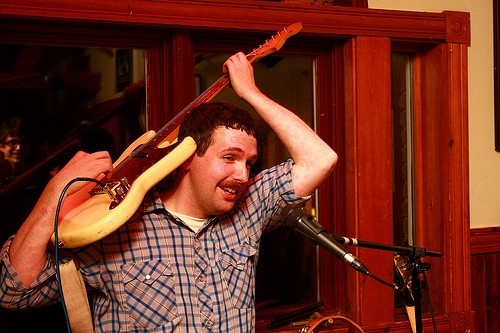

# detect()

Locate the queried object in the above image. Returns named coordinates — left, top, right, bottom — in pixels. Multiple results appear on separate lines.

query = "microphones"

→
left=280, top=204, right=373, bottom=277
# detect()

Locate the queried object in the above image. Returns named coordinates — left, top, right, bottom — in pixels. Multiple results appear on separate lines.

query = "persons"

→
left=1, top=117, right=28, bottom=163
left=0, top=52, right=338, bottom=333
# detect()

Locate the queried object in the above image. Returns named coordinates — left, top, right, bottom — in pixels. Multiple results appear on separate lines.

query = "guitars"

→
left=49, top=21, right=303, bottom=249
